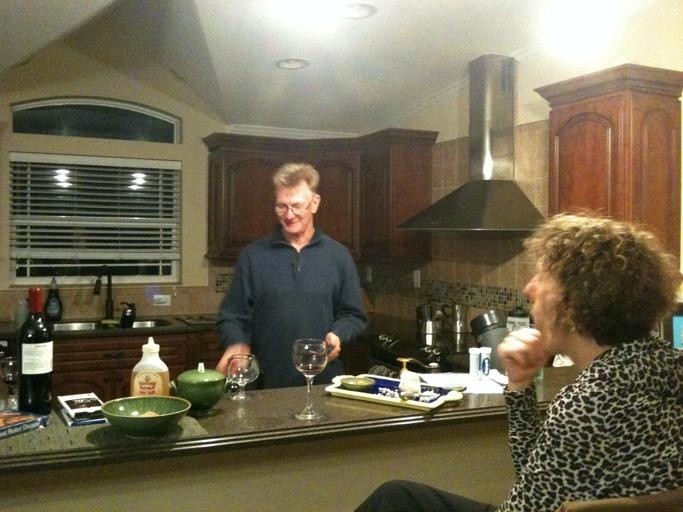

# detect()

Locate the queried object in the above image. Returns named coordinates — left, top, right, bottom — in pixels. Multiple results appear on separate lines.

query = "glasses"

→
left=274, top=195, right=315, bottom=215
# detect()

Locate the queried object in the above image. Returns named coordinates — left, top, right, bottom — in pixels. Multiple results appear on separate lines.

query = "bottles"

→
left=468, top=346, right=492, bottom=376
left=18, top=287, right=54, bottom=415
left=130, top=336, right=170, bottom=397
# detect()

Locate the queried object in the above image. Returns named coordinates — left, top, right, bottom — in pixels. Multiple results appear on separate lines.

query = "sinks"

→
left=52, top=320, right=110, bottom=331
left=107, top=319, right=172, bottom=329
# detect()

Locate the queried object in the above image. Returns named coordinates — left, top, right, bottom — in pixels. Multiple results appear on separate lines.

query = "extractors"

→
left=394, top=54, right=549, bottom=231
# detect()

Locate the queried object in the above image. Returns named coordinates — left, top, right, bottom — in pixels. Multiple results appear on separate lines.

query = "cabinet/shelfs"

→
left=203, top=127, right=438, bottom=264
left=534, top=63, right=683, bottom=275
left=52, top=332, right=221, bottom=404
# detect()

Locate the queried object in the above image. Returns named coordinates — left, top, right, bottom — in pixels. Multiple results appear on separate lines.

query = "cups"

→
left=0, top=356, right=20, bottom=386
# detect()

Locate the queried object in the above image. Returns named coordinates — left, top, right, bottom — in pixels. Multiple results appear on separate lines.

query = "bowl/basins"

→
left=340, top=377, right=376, bottom=391
left=101, top=395, right=192, bottom=439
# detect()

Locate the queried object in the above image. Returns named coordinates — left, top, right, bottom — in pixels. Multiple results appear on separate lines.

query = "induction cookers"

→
left=363, top=323, right=480, bottom=380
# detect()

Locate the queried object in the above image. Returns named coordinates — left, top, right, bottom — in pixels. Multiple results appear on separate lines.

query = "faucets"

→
left=92, top=265, right=114, bottom=319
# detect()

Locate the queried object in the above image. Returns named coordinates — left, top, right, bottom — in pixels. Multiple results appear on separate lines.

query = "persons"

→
left=353, top=210, right=683, bottom=512
left=215, top=162, right=370, bottom=391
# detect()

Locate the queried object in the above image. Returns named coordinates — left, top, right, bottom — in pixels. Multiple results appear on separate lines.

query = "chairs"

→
left=564, top=487, right=683, bottom=512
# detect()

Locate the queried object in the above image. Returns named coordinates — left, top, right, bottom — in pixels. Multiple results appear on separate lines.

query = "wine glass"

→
left=226, top=353, right=260, bottom=401
left=291, top=338, right=329, bottom=422
